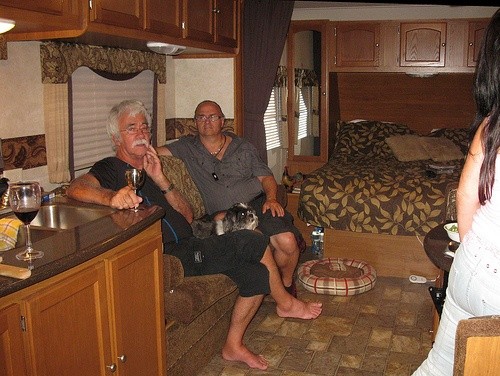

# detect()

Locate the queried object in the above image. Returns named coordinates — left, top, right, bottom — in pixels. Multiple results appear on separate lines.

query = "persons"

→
left=66, top=99, right=323, bottom=370
left=146, top=100, right=306, bottom=301
left=410, top=9, right=500, bottom=376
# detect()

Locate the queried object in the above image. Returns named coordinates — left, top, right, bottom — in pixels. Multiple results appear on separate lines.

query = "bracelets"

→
left=162, top=184, right=176, bottom=196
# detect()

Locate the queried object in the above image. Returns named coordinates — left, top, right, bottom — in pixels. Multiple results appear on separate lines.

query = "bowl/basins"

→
left=444, top=223, right=461, bottom=243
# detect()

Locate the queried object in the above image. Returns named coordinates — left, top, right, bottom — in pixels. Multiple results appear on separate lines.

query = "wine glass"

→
left=8, top=181, right=44, bottom=261
left=124, top=168, right=147, bottom=212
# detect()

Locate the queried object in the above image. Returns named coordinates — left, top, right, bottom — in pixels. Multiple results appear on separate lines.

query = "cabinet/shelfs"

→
left=0, top=194, right=171, bottom=376
left=1, top=1, right=240, bottom=60
left=326, top=17, right=494, bottom=73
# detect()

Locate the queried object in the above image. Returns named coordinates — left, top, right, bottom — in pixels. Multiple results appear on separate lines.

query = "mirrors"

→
left=288, top=18, right=330, bottom=162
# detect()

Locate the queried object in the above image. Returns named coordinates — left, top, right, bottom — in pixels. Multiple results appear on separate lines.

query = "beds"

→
left=297, top=72, right=471, bottom=276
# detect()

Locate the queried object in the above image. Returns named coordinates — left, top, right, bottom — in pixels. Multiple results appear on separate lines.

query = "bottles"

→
left=310, top=226, right=324, bottom=259
left=281, top=167, right=290, bottom=188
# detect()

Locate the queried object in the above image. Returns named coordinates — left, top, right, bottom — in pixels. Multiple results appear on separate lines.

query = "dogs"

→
left=189, top=202, right=259, bottom=239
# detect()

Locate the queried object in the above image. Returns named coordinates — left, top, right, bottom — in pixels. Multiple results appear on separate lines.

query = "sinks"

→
left=0, top=222, right=60, bottom=259
left=1, top=201, right=116, bottom=230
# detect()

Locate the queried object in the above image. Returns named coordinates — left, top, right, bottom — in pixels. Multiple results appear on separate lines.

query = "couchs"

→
left=158, top=245, right=238, bottom=376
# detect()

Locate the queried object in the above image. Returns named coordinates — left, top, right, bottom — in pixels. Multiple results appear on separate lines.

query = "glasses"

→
left=119, top=125, right=152, bottom=135
left=194, top=115, right=221, bottom=122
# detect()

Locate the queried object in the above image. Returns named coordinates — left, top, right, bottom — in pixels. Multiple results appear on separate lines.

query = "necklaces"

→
left=201, top=135, right=227, bottom=160
